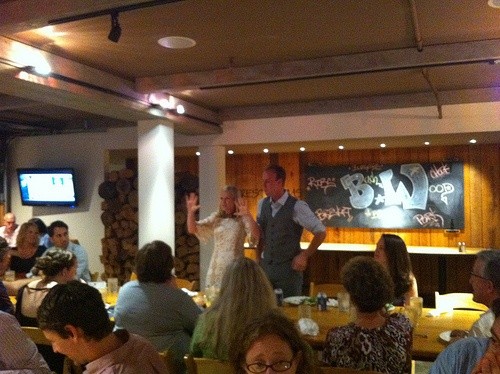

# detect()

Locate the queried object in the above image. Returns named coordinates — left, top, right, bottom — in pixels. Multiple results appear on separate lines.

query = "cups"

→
left=337, top=293, right=350, bottom=313
left=409, top=297, right=423, bottom=317
left=459, top=241, right=466, bottom=252
left=403, top=305, right=420, bottom=327
left=5, top=271, right=15, bottom=282
left=107, top=278, right=118, bottom=294
left=298, top=305, right=311, bottom=320
left=203, top=287, right=219, bottom=308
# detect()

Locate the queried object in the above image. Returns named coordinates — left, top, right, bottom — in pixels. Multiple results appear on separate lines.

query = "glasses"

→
left=468, top=269, right=489, bottom=282
left=242, top=355, right=296, bottom=374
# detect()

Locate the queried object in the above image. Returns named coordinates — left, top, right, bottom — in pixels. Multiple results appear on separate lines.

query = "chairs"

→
left=18, top=271, right=492, bottom=374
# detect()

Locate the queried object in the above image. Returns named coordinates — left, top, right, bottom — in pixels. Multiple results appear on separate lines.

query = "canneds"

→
left=459, top=242, right=466, bottom=252
left=317, top=292, right=327, bottom=311
left=274, top=288, right=283, bottom=306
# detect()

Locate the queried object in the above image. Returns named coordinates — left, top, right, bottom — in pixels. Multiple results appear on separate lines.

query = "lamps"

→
left=108, top=14, right=125, bottom=44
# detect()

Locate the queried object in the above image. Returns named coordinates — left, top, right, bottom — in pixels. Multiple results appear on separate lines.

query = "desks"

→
left=3, top=275, right=209, bottom=323
left=250, top=241, right=489, bottom=292
left=200, top=293, right=488, bottom=361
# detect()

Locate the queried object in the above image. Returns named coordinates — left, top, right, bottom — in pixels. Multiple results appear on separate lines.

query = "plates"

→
left=440, top=331, right=469, bottom=342
left=283, top=296, right=310, bottom=305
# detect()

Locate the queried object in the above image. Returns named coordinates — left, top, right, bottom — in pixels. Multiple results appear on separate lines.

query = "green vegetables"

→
left=301, top=296, right=318, bottom=306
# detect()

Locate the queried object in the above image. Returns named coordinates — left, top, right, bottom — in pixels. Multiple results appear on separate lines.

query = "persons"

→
left=36, top=280, right=172, bottom=374
left=321, top=255, right=413, bottom=374
left=113, top=240, right=204, bottom=358
left=374, top=234, right=419, bottom=307
left=185, top=184, right=261, bottom=299
left=0, top=213, right=92, bottom=374
left=255, top=164, right=327, bottom=299
left=229, top=317, right=324, bottom=374
left=187, top=257, right=281, bottom=364
left=425, top=249, right=500, bottom=374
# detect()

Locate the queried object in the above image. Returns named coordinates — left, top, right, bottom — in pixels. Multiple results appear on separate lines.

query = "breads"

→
left=449, top=329, right=467, bottom=343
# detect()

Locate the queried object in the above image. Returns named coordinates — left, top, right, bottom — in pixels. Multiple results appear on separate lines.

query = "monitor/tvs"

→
left=16, top=167, right=78, bottom=207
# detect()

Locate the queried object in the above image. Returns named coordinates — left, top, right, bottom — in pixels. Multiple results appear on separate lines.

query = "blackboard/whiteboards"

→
left=305, top=159, right=465, bottom=229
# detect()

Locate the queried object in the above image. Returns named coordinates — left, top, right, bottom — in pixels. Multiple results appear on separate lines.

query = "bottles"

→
left=317, top=292, right=328, bottom=311
left=274, top=288, right=283, bottom=307
